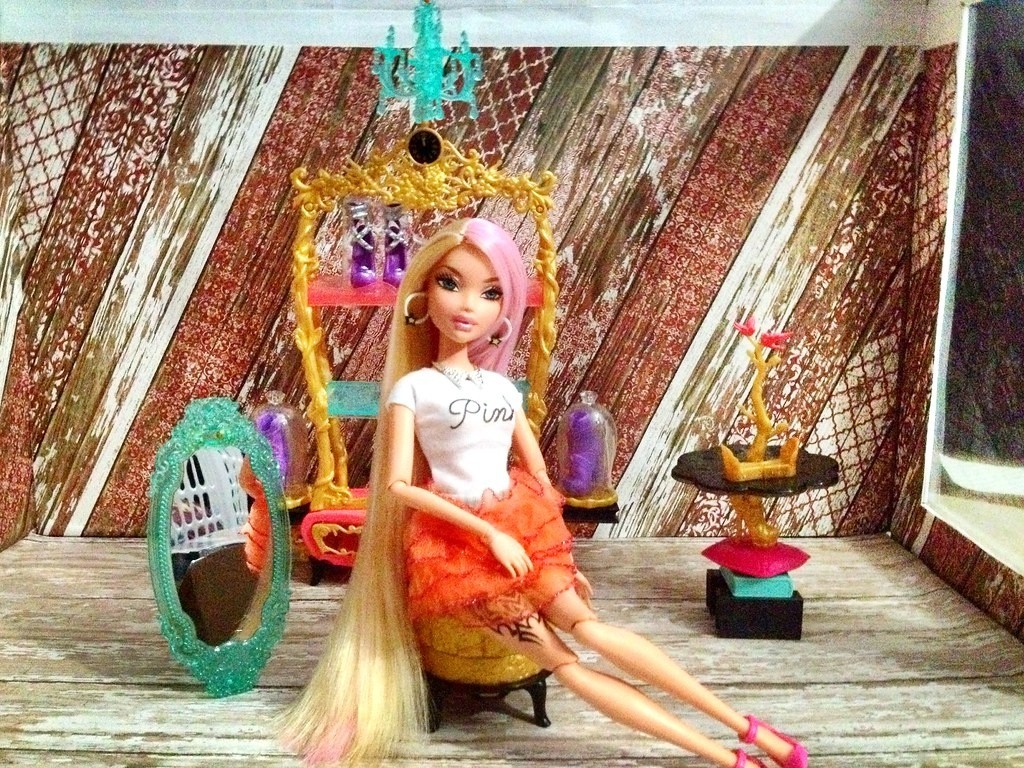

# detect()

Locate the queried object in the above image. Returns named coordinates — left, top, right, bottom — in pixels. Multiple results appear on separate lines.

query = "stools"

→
left=411, top=614, right=557, bottom=732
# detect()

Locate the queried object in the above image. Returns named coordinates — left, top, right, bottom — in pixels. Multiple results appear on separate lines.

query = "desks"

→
left=670, top=445, right=839, bottom=639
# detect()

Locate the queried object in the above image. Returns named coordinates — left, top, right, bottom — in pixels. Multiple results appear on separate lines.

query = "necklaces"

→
left=431, top=360, right=484, bottom=389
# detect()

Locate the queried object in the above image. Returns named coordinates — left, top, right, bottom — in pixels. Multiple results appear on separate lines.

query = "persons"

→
left=270, top=217, right=807, bottom=768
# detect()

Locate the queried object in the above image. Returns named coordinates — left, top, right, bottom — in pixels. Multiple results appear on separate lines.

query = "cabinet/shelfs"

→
left=292, top=126, right=566, bottom=585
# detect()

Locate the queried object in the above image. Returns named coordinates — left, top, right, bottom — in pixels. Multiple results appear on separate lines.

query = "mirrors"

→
left=139, top=393, right=301, bottom=702
